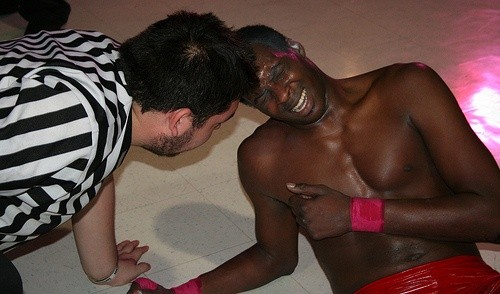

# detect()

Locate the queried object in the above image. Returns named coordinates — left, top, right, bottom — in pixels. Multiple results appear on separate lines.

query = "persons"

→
left=0, top=9, right=263, bottom=294
left=125, top=22, right=499, bottom=293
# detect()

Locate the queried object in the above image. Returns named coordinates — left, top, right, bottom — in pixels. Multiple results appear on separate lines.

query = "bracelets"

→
left=349, top=196, right=385, bottom=234
left=86, top=262, right=120, bottom=286
left=169, top=277, right=203, bottom=293
